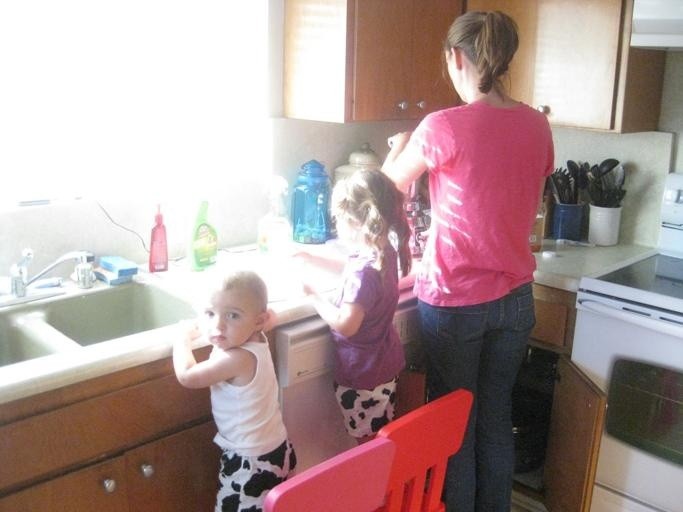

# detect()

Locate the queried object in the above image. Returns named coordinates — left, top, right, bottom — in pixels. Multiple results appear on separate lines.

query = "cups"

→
left=549, top=195, right=586, bottom=249
left=586, top=196, right=624, bottom=249
left=148, top=203, right=169, bottom=274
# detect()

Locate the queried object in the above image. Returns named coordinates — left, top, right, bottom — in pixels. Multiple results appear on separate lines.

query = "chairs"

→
left=375, top=388, right=474, bottom=512
left=262, top=437, right=395, bottom=512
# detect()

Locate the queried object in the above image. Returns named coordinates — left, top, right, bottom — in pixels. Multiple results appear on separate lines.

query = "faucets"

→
left=10, top=248, right=95, bottom=297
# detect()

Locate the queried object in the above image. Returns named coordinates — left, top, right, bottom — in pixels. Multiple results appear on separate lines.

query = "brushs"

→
left=556, top=239, right=596, bottom=248
left=615, top=165, right=625, bottom=206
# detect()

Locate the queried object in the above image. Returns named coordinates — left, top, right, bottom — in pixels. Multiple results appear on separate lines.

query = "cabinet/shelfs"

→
left=284, top=0, right=466, bottom=123
left=0, top=331, right=280, bottom=512
left=509, top=284, right=611, bottom=512
left=466, top=0, right=667, bottom=133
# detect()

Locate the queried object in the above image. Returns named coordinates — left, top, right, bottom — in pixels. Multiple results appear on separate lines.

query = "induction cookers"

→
left=578, top=246, right=682, bottom=323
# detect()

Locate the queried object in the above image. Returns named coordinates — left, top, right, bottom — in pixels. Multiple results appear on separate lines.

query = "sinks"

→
left=0, top=311, right=83, bottom=408
left=41, top=282, right=223, bottom=379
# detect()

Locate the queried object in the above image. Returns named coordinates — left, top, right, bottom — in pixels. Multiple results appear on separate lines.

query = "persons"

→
left=377, top=8, right=558, bottom=511
left=171, top=270, right=298, bottom=512
left=294, top=165, right=414, bottom=446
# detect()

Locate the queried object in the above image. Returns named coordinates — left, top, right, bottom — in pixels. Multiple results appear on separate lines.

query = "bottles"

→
left=191, top=201, right=218, bottom=271
left=333, top=142, right=387, bottom=187
left=289, top=152, right=332, bottom=247
left=526, top=201, right=548, bottom=253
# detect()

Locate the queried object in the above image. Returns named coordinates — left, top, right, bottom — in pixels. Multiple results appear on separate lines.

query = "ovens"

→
left=570, top=292, right=682, bottom=511
